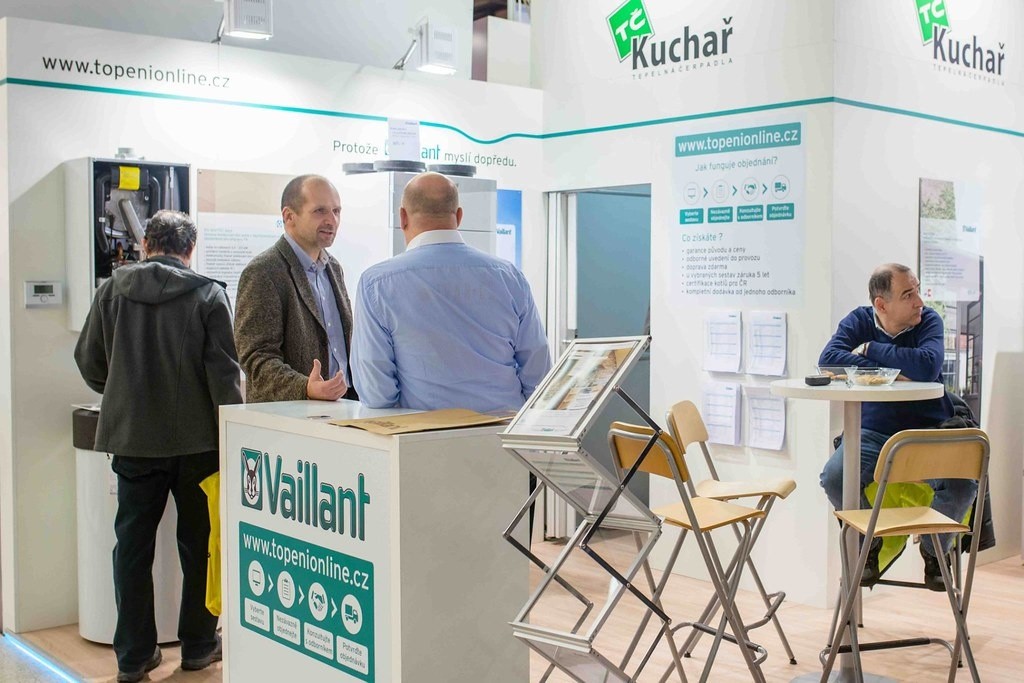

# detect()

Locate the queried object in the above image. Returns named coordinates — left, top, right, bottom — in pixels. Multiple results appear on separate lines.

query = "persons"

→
left=72, top=207, right=244, bottom=683
left=349, top=169, right=552, bottom=552
left=819, top=264, right=976, bottom=592
left=231, top=173, right=354, bottom=402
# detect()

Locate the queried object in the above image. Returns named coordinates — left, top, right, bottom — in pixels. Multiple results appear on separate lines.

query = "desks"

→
left=771, top=377, right=945, bottom=683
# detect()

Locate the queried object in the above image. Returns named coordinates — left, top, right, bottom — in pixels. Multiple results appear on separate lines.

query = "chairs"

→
left=663, top=399, right=797, bottom=683
left=607, top=423, right=768, bottom=683
left=818, top=427, right=991, bottom=683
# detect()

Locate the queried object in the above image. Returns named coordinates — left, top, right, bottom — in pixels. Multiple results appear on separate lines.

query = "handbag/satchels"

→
left=198, top=470, right=222, bottom=617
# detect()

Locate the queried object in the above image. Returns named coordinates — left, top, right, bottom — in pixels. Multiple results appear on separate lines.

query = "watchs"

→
left=857, top=342, right=865, bottom=356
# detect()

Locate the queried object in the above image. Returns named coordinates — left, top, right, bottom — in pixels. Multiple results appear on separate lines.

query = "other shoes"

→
left=117, top=645, right=162, bottom=682
left=181, top=633, right=222, bottom=670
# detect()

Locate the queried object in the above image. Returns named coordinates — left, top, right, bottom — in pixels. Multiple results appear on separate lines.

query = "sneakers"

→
left=860, top=549, right=880, bottom=586
left=920, top=542, right=953, bottom=591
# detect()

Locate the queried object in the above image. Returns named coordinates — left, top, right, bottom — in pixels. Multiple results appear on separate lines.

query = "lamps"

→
left=211, top=0, right=274, bottom=45
left=389, top=14, right=457, bottom=75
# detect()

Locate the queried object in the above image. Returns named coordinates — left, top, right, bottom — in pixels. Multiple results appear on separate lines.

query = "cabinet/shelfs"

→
left=330, top=171, right=497, bottom=307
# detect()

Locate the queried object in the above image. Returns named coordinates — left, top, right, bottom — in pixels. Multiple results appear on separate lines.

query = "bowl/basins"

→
left=844, top=367, right=901, bottom=386
left=814, top=364, right=858, bottom=383
